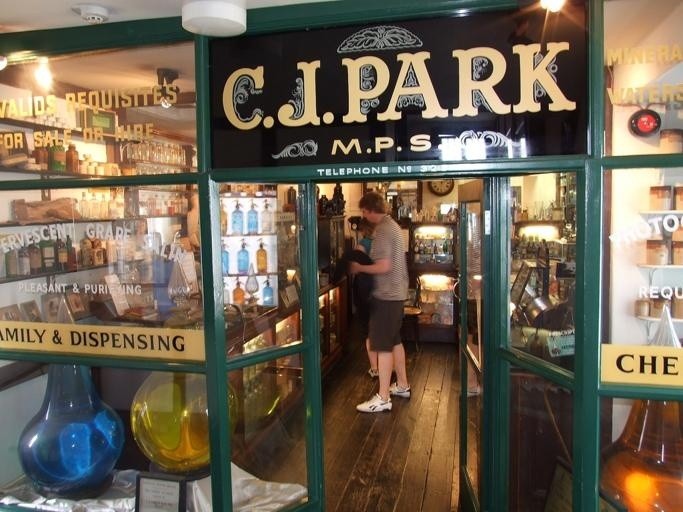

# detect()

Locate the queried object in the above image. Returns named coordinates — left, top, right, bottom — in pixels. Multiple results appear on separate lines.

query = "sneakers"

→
left=355, top=392, right=393, bottom=414
left=367, top=367, right=379, bottom=379
left=388, top=381, right=411, bottom=399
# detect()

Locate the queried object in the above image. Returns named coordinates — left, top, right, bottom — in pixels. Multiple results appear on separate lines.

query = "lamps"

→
left=182, top=2, right=246, bottom=36
left=154, top=68, right=179, bottom=110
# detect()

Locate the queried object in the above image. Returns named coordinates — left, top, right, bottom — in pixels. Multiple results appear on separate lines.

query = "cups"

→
left=557, top=282, right=570, bottom=301
left=135, top=164, right=181, bottom=175
left=634, top=128, right=683, bottom=320
left=77, top=159, right=120, bottom=177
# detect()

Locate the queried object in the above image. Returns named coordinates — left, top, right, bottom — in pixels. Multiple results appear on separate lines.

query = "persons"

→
left=348, top=192, right=413, bottom=414
left=338, top=217, right=381, bottom=380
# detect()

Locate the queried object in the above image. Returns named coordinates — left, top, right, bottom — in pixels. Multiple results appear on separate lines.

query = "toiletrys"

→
left=218, top=196, right=275, bottom=307
left=511, top=198, right=562, bottom=265
left=409, top=202, right=454, bottom=264
left=4, top=137, right=191, bottom=281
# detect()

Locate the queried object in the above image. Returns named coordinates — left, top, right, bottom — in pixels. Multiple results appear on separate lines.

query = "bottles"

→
left=409, top=203, right=457, bottom=222
left=5, top=231, right=72, bottom=279
left=80, top=231, right=106, bottom=266
left=512, top=199, right=551, bottom=221
left=126, top=137, right=185, bottom=165
left=135, top=191, right=191, bottom=217
left=512, top=233, right=560, bottom=258
left=65, top=144, right=79, bottom=174
left=414, top=240, right=454, bottom=257
left=78, top=190, right=123, bottom=220
